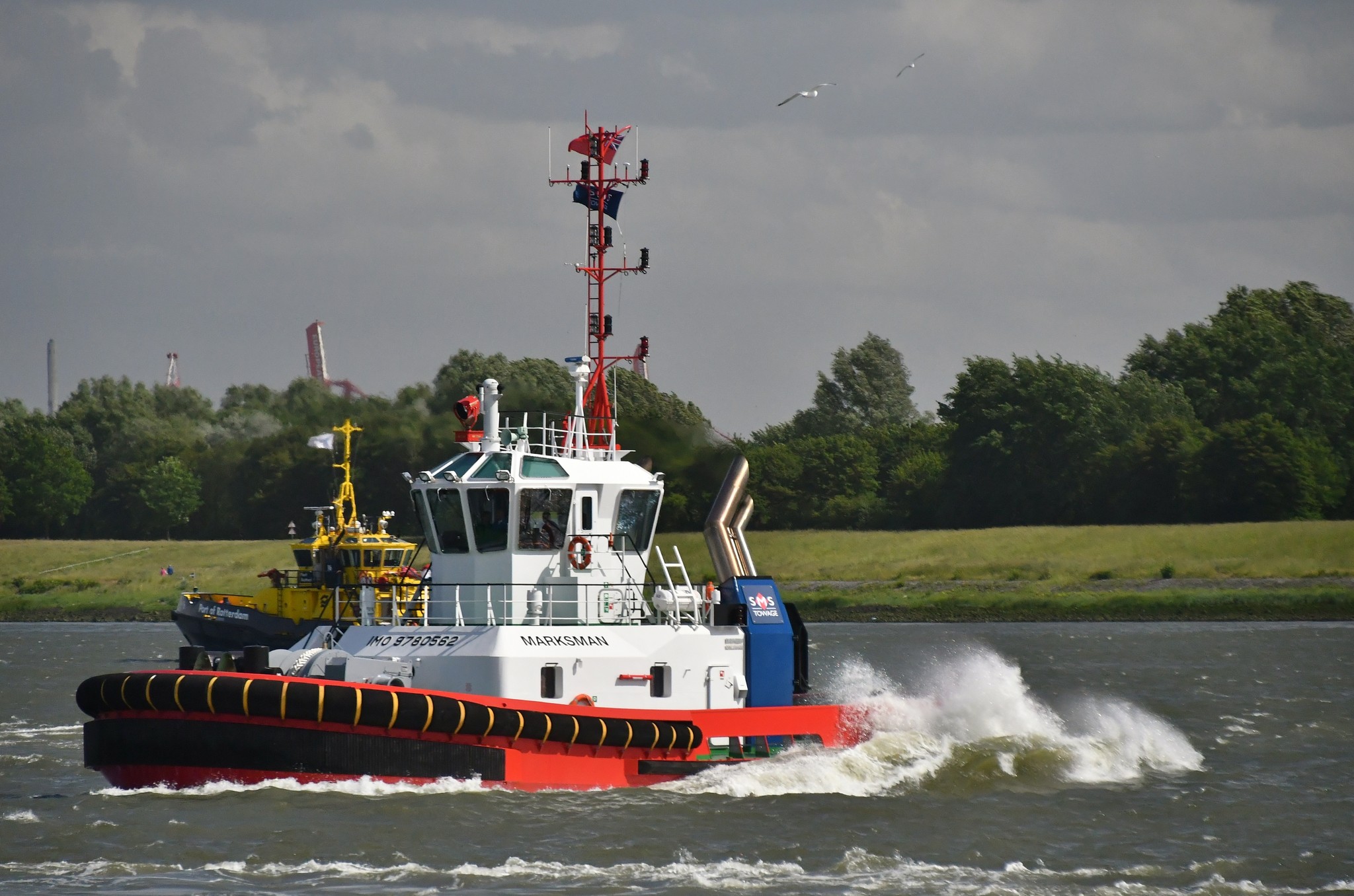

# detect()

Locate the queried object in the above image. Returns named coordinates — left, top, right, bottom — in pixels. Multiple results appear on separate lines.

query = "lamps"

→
left=653, top=472, right=666, bottom=482
left=640, top=247, right=649, bottom=268
left=444, top=471, right=461, bottom=483
left=589, top=315, right=599, bottom=333
left=418, top=471, right=433, bottom=482
left=312, top=510, right=323, bottom=535
left=604, top=225, right=613, bottom=245
left=401, top=472, right=414, bottom=483
left=603, top=314, right=613, bottom=334
left=640, top=158, right=649, bottom=177
left=590, top=135, right=598, bottom=156
left=589, top=225, right=599, bottom=245
left=495, top=469, right=514, bottom=482
left=453, top=395, right=484, bottom=442
left=581, top=160, right=588, bottom=178
left=640, top=335, right=649, bottom=355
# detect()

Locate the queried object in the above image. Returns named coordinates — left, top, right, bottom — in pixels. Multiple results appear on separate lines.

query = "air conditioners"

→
left=378, top=520, right=388, bottom=534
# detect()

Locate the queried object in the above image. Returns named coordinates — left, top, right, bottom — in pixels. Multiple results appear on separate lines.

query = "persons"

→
left=167, top=565, right=173, bottom=575
left=160, top=567, right=166, bottom=576
left=541, top=512, right=560, bottom=541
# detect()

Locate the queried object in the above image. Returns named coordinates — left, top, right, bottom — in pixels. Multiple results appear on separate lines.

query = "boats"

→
left=169, top=412, right=428, bottom=648
left=74, top=104, right=872, bottom=787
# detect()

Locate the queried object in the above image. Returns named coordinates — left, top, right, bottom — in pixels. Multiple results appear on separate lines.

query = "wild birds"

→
left=896, top=51, right=926, bottom=79
left=776, top=81, right=839, bottom=108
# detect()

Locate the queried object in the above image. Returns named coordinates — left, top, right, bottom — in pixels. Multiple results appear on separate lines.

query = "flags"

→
left=307, top=433, right=335, bottom=450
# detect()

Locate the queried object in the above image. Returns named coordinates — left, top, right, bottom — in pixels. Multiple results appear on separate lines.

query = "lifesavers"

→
left=570, top=694, right=594, bottom=707
left=378, top=576, right=389, bottom=584
left=706, top=581, right=714, bottom=611
left=359, top=570, right=367, bottom=583
left=568, top=537, right=591, bottom=569
left=409, top=622, right=417, bottom=626
